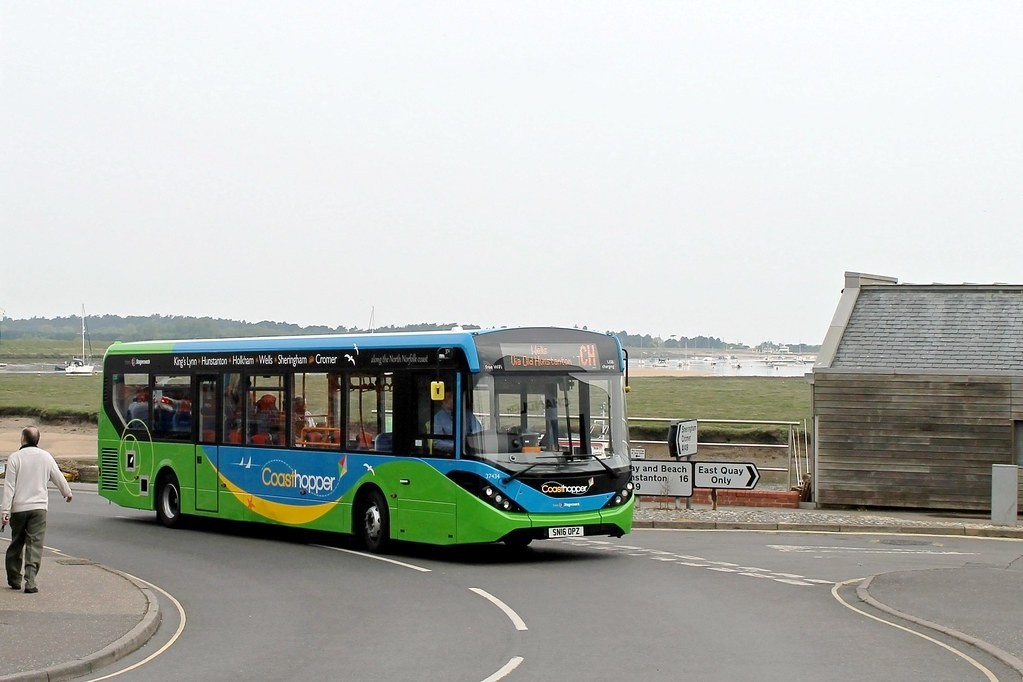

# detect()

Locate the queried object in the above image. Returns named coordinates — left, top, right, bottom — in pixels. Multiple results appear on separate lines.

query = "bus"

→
left=97, top=324, right=636, bottom=555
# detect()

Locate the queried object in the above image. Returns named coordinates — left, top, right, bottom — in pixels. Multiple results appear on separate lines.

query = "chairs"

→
left=326, top=432, right=340, bottom=448
left=249, top=432, right=273, bottom=445
left=305, top=432, right=323, bottom=449
left=203, top=429, right=216, bottom=441
left=356, top=431, right=374, bottom=450
left=226, top=431, right=241, bottom=444
left=154, top=403, right=173, bottom=429
left=173, top=399, right=191, bottom=432
left=374, top=433, right=392, bottom=452
left=131, top=402, right=149, bottom=430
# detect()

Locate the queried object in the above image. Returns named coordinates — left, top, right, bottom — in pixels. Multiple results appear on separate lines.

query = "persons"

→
left=434, top=387, right=482, bottom=453
left=2, top=426, right=72, bottom=593
left=293, top=396, right=316, bottom=447
left=225, top=379, right=281, bottom=445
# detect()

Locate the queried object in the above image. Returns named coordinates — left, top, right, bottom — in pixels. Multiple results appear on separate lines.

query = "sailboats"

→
left=63, top=303, right=104, bottom=376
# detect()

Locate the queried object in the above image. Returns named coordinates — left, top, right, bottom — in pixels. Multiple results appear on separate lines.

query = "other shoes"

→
left=24, top=586, right=39, bottom=593
left=12, top=585, right=21, bottom=589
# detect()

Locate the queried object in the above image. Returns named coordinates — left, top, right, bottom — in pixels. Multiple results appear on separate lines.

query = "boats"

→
left=635, top=332, right=817, bottom=372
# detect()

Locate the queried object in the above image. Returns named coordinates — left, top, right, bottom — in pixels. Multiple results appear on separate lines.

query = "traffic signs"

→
left=676, top=420, right=698, bottom=458
left=694, top=460, right=760, bottom=489
